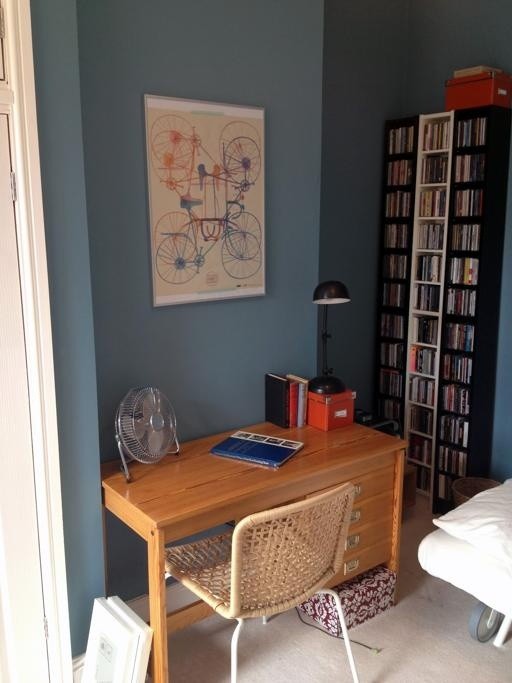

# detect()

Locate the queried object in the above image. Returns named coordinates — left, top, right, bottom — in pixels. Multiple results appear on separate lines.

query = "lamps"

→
left=307, top=280, right=352, bottom=393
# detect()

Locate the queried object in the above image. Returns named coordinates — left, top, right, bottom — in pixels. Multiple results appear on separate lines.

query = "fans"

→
left=113, top=385, right=180, bottom=483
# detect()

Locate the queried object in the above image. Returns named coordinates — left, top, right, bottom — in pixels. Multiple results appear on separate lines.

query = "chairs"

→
left=165, top=479, right=360, bottom=683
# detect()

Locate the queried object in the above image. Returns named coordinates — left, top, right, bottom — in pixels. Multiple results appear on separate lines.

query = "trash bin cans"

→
left=450, top=477, right=501, bottom=507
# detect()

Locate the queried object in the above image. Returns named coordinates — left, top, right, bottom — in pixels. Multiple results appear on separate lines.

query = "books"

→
left=264, top=372, right=309, bottom=429
left=208, top=429, right=305, bottom=467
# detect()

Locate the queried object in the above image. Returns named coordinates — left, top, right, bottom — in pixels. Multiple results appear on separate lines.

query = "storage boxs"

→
left=296, top=562, right=398, bottom=637
left=443, top=70, right=511, bottom=111
left=305, top=388, right=357, bottom=432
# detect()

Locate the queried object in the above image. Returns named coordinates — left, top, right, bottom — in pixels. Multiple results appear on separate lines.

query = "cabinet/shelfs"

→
left=369, top=102, right=512, bottom=514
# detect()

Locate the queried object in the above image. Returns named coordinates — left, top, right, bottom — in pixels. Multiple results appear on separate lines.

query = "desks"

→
left=101, top=420, right=408, bottom=683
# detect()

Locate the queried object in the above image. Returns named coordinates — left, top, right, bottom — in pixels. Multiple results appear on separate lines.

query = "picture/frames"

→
left=143, top=93, right=267, bottom=308
